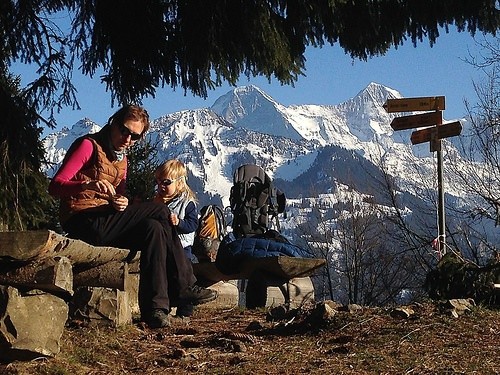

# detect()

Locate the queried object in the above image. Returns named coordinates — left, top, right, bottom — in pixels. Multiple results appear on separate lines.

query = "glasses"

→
left=118, top=123, right=140, bottom=141
left=156, top=176, right=184, bottom=186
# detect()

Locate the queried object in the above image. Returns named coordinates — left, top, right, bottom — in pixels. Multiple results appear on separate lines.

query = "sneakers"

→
left=179, top=286, right=217, bottom=304
left=144, top=309, right=170, bottom=328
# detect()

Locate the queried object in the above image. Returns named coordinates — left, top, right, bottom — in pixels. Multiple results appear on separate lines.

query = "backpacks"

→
left=195, top=205, right=226, bottom=259
left=229, top=164, right=286, bottom=240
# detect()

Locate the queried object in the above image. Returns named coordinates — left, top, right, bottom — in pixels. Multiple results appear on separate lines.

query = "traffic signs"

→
left=390, top=111, right=442, bottom=131
left=382, top=96, right=445, bottom=113
left=410, top=121, right=462, bottom=145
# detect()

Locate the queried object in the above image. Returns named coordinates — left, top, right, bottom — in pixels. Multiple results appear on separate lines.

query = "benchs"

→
left=0, top=230, right=141, bottom=357
left=177, top=256, right=326, bottom=306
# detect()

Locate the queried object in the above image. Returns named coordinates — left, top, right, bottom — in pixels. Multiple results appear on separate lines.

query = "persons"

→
left=48, top=104, right=218, bottom=329
left=153, top=158, right=200, bottom=318
